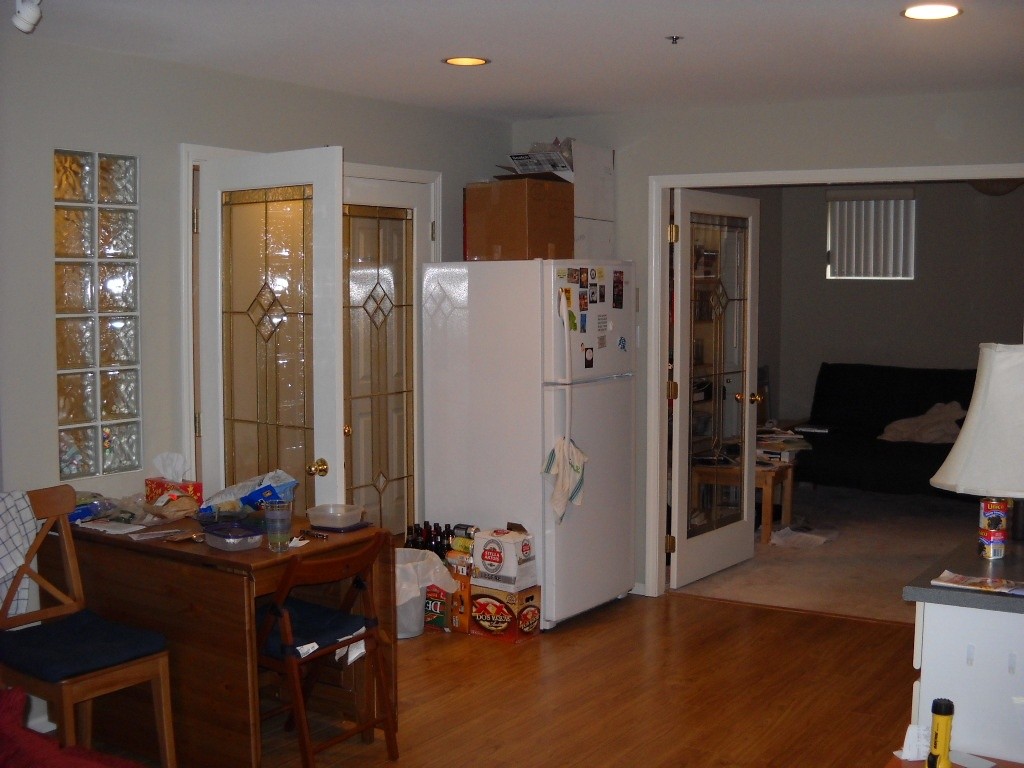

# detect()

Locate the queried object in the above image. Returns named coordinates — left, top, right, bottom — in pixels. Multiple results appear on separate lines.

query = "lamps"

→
left=11, top=0, right=42, bottom=33
left=929, top=343, right=1024, bottom=499
left=966, top=177, right=1024, bottom=196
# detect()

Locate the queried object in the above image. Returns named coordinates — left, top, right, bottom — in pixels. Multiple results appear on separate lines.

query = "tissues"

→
left=144, top=452, right=204, bottom=512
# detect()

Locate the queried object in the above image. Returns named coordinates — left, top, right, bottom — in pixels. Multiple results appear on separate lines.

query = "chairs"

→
left=255, top=530, right=399, bottom=768
left=1, top=485, right=178, bottom=768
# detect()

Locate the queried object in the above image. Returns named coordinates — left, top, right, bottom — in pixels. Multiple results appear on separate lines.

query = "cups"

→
left=264, top=500, right=292, bottom=552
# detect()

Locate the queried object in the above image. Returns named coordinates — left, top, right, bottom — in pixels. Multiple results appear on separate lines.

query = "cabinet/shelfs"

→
left=668, top=223, right=721, bottom=453
left=902, top=538, right=1024, bottom=762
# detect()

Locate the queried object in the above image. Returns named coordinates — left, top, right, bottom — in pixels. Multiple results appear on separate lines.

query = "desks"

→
left=36, top=512, right=396, bottom=768
left=690, top=459, right=799, bottom=544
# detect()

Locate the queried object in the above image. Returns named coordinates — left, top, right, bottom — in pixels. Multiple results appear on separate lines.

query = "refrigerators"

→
left=421, top=257, right=636, bottom=631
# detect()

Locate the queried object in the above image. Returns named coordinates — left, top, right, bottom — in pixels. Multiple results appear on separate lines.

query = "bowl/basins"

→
left=305, top=503, right=365, bottom=528
left=202, top=522, right=264, bottom=551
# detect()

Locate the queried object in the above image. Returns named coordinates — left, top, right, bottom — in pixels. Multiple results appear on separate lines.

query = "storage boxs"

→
left=563, top=141, right=615, bottom=260
left=466, top=172, right=574, bottom=261
left=424, top=522, right=542, bottom=645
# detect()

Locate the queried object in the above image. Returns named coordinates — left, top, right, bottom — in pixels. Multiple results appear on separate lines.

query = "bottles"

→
left=404, top=521, right=455, bottom=561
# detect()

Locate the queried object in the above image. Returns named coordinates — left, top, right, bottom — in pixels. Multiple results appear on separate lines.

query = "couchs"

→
left=785, top=362, right=978, bottom=500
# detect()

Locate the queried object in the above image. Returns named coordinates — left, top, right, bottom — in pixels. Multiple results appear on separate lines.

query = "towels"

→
left=541, top=434, right=588, bottom=528
